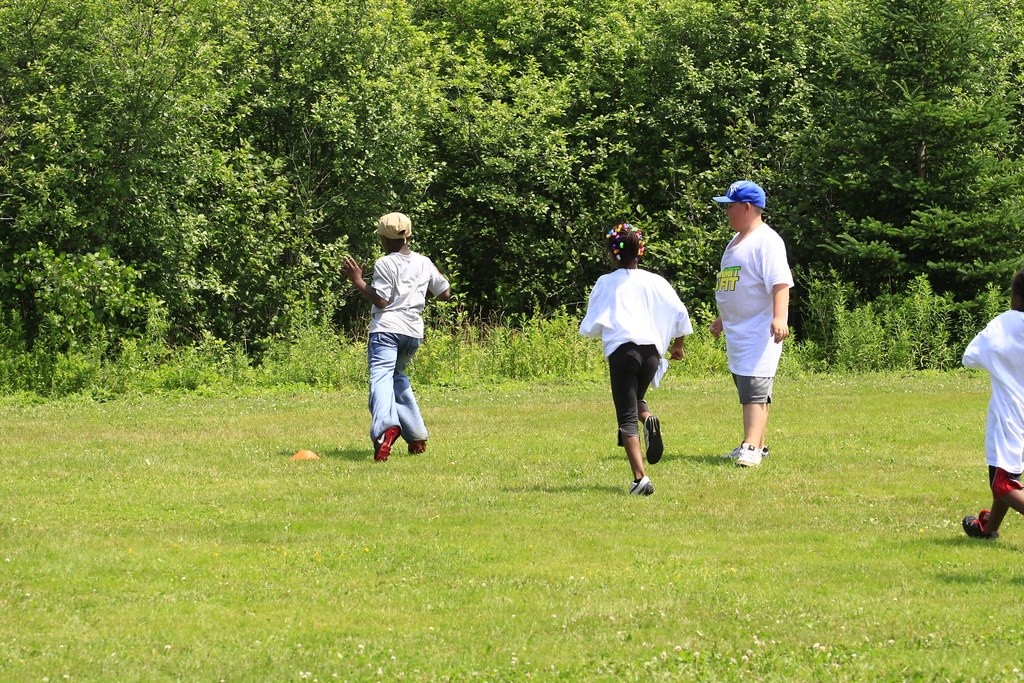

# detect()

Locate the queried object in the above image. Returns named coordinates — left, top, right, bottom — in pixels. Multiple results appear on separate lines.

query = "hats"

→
left=378, top=212, right=412, bottom=239
left=712, top=181, right=767, bottom=209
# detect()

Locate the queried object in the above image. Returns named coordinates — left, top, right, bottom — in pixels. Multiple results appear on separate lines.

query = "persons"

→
left=342, top=212, right=450, bottom=461
left=578, top=223, right=693, bottom=497
left=961, top=267, right=1024, bottom=540
left=707, top=180, right=796, bottom=469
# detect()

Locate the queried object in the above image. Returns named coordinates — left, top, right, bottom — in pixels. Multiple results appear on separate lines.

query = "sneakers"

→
left=644, top=416, right=665, bottom=465
left=373, top=425, right=400, bottom=461
left=628, top=477, right=653, bottom=497
left=720, top=443, right=770, bottom=467
left=408, top=438, right=427, bottom=454
left=963, top=511, right=999, bottom=540
